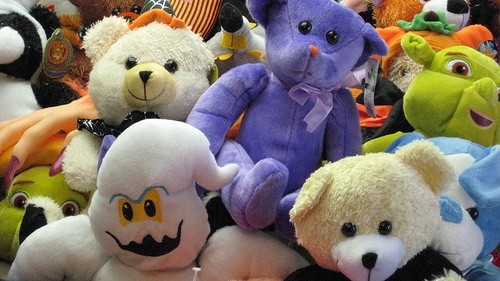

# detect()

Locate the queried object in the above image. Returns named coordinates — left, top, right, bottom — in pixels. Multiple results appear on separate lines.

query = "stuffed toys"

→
left=0, top=0, right=500, bottom=281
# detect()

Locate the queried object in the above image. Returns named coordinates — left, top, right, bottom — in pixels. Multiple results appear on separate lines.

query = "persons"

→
left=0, top=93, right=97, bottom=186
